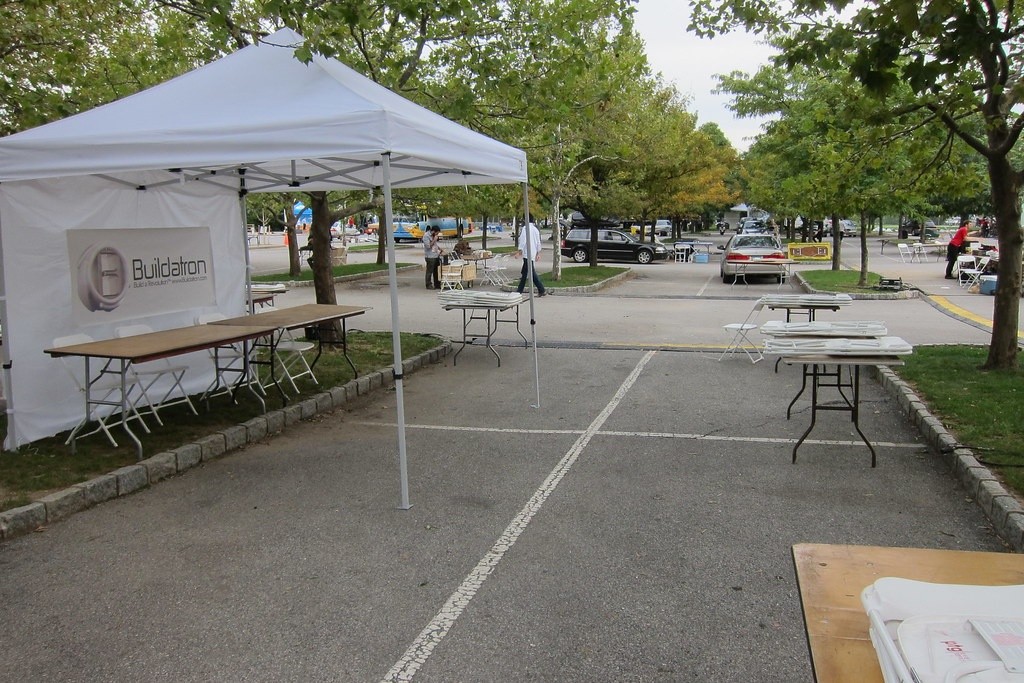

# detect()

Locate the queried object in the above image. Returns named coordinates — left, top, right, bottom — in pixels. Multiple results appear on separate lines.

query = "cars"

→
left=716, top=233, right=787, bottom=284
left=329, top=217, right=381, bottom=238
left=623, top=219, right=674, bottom=238
left=829, top=220, right=857, bottom=237
left=901, top=215, right=938, bottom=236
left=735, top=216, right=783, bottom=247
left=797, top=219, right=832, bottom=237
left=538, top=218, right=564, bottom=228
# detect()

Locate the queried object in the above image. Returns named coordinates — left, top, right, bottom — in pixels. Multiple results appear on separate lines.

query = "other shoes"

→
left=533, top=291, right=546, bottom=297
left=945, top=274, right=957, bottom=279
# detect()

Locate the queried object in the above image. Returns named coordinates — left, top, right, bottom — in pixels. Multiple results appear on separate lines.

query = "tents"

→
left=0, top=26, right=541, bottom=509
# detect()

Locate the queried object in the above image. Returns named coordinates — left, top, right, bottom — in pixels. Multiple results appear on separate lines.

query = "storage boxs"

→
left=438, top=265, right=476, bottom=281
left=695, top=254, right=709, bottom=263
left=980, top=275, right=997, bottom=295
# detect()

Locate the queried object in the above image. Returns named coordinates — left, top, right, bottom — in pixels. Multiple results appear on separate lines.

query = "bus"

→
left=391, top=196, right=473, bottom=244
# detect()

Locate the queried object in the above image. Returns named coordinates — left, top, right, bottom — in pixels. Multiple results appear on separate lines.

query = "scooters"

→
left=978, top=220, right=999, bottom=238
left=718, top=224, right=726, bottom=235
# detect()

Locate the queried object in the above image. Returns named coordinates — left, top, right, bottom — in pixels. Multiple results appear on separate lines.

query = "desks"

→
left=727, top=261, right=800, bottom=295
left=780, top=336, right=905, bottom=468
left=418, top=235, right=451, bottom=248
left=299, top=249, right=312, bottom=265
left=441, top=296, right=530, bottom=367
left=453, top=257, right=493, bottom=288
left=675, top=241, right=713, bottom=263
left=766, top=294, right=851, bottom=373
left=44, top=290, right=373, bottom=458
left=908, top=244, right=948, bottom=263
left=791, top=541, right=1024, bottom=683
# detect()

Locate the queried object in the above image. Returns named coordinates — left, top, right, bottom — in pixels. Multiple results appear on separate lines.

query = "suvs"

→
left=561, top=209, right=669, bottom=264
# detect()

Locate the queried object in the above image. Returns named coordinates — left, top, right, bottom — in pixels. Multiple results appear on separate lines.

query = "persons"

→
left=944, top=220, right=972, bottom=279
left=979, top=216, right=990, bottom=237
left=839, top=218, right=846, bottom=243
left=423, top=225, right=444, bottom=290
left=515, top=212, right=547, bottom=298
left=812, top=222, right=823, bottom=242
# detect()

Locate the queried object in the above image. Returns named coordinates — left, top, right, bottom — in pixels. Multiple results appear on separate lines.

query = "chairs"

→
left=195, top=313, right=267, bottom=404
left=53, top=333, right=151, bottom=447
left=898, top=243, right=928, bottom=263
left=861, top=576, right=1024, bottom=683
left=718, top=297, right=912, bottom=366
left=115, top=324, right=198, bottom=426
left=675, top=245, right=703, bottom=263
left=437, top=289, right=523, bottom=309
left=441, top=260, right=464, bottom=292
left=479, top=254, right=510, bottom=287
left=957, top=256, right=990, bottom=289
left=257, top=307, right=318, bottom=394
